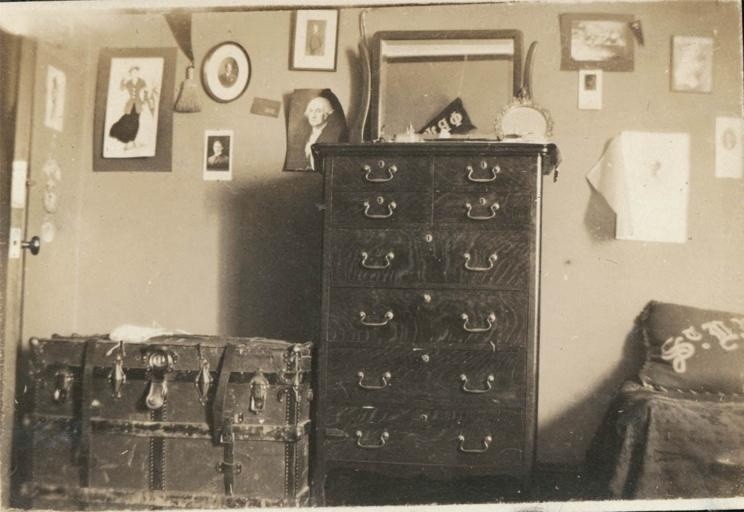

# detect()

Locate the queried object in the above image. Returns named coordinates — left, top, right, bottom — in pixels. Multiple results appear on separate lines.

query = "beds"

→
left=603, top=380, right=744, bottom=497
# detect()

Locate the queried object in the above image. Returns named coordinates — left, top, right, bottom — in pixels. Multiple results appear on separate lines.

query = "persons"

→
left=288, top=97, right=340, bottom=175
left=206, top=139, right=229, bottom=170
left=108, top=64, right=155, bottom=151
left=307, top=23, right=323, bottom=54
left=219, top=62, right=238, bottom=88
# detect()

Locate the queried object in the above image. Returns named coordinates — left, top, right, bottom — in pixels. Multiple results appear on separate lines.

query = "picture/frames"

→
left=199, top=41, right=251, bottom=104
left=288, top=8, right=340, bottom=72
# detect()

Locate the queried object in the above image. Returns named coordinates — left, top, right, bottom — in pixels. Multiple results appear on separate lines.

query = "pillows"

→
left=635, top=299, right=744, bottom=402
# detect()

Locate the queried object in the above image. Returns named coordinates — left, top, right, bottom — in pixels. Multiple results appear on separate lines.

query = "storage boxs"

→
left=8, top=334, right=309, bottom=510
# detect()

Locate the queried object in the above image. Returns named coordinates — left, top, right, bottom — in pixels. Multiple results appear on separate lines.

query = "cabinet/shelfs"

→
left=308, top=143, right=547, bottom=508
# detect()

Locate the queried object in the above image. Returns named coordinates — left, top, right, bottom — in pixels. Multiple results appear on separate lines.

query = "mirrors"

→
left=374, top=30, right=523, bottom=141
